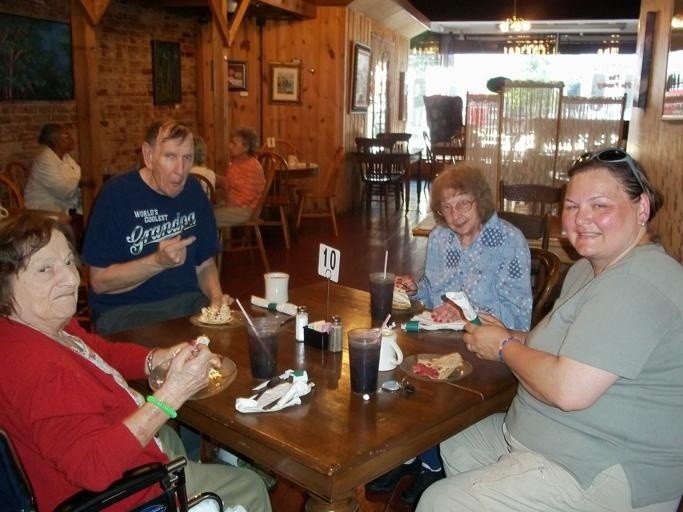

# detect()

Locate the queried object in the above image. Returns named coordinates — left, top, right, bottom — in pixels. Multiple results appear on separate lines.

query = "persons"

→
left=1, top=209, right=272, bottom=511
left=25, top=122, right=94, bottom=231
left=369, top=165, right=534, bottom=502
left=417, top=149, right=683, bottom=512
left=193, top=135, right=216, bottom=200
left=82, top=119, right=275, bottom=492
left=211, top=127, right=268, bottom=229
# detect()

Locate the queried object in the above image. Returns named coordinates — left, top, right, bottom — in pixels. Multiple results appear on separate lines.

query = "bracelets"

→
left=148, top=347, right=158, bottom=371
left=499, top=337, right=514, bottom=364
left=147, top=393, right=177, bottom=421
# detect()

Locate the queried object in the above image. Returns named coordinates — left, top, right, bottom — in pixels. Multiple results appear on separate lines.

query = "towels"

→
left=410, top=310, right=467, bottom=332
left=234, top=368, right=312, bottom=413
left=249, top=295, right=298, bottom=317
left=444, top=291, right=476, bottom=321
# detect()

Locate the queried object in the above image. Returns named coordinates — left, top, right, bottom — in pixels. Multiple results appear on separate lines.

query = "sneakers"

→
left=400, top=464, right=446, bottom=504
left=214, top=447, right=277, bottom=489
left=366, top=457, right=420, bottom=493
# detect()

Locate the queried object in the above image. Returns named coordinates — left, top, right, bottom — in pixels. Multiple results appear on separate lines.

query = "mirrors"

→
left=661, top=0, right=683, bottom=121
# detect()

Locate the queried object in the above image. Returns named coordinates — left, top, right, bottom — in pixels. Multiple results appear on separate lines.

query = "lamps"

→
left=498, top=0, right=532, bottom=35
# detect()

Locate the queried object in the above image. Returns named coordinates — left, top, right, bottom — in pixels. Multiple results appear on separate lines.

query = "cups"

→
left=264, top=273, right=289, bottom=304
left=248, top=317, right=285, bottom=376
left=347, top=329, right=382, bottom=392
left=370, top=271, right=395, bottom=322
left=289, top=155, right=300, bottom=167
left=375, top=326, right=403, bottom=370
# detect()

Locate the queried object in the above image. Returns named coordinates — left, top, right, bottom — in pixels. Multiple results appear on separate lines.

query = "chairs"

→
left=499, top=180, right=568, bottom=237
left=6, top=161, right=28, bottom=210
left=529, top=246, right=561, bottom=330
left=1, top=174, right=76, bottom=248
left=496, top=211, right=552, bottom=252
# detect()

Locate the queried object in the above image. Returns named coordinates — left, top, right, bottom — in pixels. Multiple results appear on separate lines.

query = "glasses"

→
left=571, top=149, right=644, bottom=190
left=436, top=199, right=479, bottom=217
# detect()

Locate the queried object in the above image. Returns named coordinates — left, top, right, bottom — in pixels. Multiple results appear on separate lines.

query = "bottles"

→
left=329, top=317, right=344, bottom=354
left=296, top=305, right=309, bottom=343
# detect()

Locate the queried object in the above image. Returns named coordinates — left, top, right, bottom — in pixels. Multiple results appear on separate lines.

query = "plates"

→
left=191, top=310, right=246, bottom=329
left=391, top=299, right=424, bottom=317
left=147, top=355, right=236, bottom=400
left=400, top=354, right=473, bottom=383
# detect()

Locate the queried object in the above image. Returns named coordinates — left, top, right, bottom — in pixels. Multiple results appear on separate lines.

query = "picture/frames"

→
left=268, top=62, right=302, bottom=105
left=632, top=12, right=657, bottom=110
left=151, top=40, right=182, bottom=105
left=228, top=60, right=247, bottom=92
left=347, top=41, right=374, bottom=113
left=0, top=12, right=75, bottom=102
left=398, top=71, right=409, bottom=122
